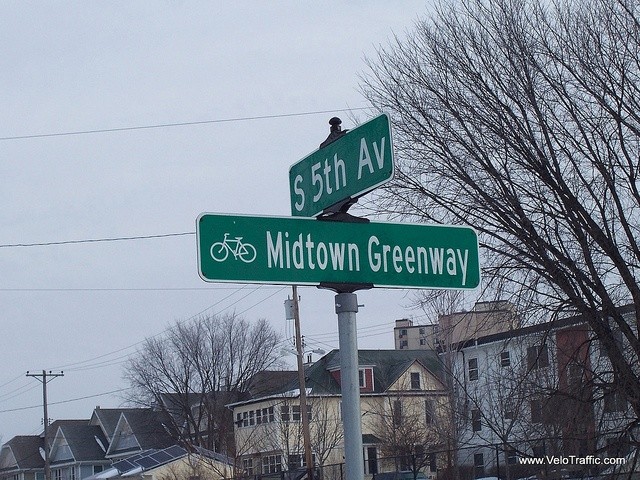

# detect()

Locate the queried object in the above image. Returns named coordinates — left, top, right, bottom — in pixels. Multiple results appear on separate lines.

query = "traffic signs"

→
left=288, top=111, right=395, bottom=217
left=196, top=211, right=481, bottom=290
